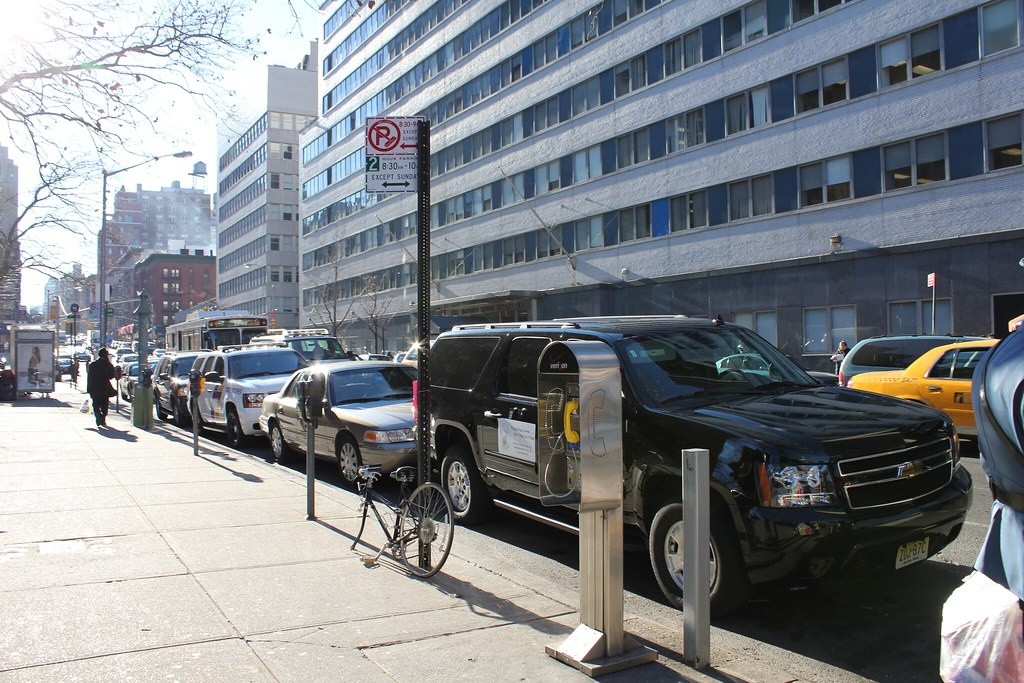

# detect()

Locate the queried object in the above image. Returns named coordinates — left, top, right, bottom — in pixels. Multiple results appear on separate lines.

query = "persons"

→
left=86, top=347, right=117, bottom=426
left=28, top=347, right=49, bottom=386
left=830, top=341, right=852, bottom=375
left=987, top=333, right=997, bottom=339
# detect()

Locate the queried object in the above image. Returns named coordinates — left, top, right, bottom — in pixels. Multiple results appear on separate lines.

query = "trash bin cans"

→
left=0, top=370, right=16, bottom=400
left=133, top=382, right=153, bottom=428
left=54, top=363, right=61, bottom=382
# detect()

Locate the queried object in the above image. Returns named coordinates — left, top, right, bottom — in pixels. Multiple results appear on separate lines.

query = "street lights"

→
left=98, top=149, right=193, bottom=354
left=243, top=263, right=276, bottom=329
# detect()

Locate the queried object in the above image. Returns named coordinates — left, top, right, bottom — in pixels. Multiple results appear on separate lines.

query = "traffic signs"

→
left=364, top=155, right=420, bottom=195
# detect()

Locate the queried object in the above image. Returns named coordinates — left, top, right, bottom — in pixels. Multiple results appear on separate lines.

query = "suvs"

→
left=358, top=338, right=437, bottom=366
left=836, top=334, right=996, bottom=390
left=151, top=353, right=203, bottom=428
left=413, top=314, right=975, bottom=627
left=189, top=344, right=311, bottom=449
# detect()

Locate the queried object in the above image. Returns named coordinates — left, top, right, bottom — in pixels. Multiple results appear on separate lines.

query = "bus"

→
left=166, top=308, right=268, bottom=352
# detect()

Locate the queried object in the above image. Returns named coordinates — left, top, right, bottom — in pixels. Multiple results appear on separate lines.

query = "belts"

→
left=987, top=476, right=1024, bottom=513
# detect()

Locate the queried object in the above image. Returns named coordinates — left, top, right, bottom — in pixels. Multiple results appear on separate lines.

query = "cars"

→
left=55, top=327, right=167, bottom=371
left=843, top=338, right=1003, bottom=446
left=55, top=353, right=79, bottom=381
left=713, top=355, right=839, bottom=391
left=122, top=361, right=156, bottom=402
left=259, top=360, right=419, bottom=486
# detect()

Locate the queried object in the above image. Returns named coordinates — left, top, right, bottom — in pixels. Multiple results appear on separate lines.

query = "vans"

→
left=250, top=327, right=363, bottom=366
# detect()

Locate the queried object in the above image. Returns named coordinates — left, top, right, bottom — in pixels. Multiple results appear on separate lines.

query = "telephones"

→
left=564, top=401, right=579, bottom=443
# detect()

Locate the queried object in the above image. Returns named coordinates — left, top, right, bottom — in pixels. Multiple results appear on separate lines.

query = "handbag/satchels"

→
left=79, top=394, right=92, bottom=415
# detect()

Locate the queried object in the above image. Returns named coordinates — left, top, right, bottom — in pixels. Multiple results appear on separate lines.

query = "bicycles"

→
left=348, top=464, right=455, bottom=580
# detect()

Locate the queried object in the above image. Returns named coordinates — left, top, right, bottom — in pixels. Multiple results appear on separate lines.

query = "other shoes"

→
left=96, top=421, right=106, bottom=427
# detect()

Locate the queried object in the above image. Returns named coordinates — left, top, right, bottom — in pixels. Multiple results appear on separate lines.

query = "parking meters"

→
left=294, top=372, right=328, bottom=521
left=190, top=368, right=200, bottom=455
left=114, top=365, right=122, bottom=413
left=141, top=369, right=154, bottom=430
